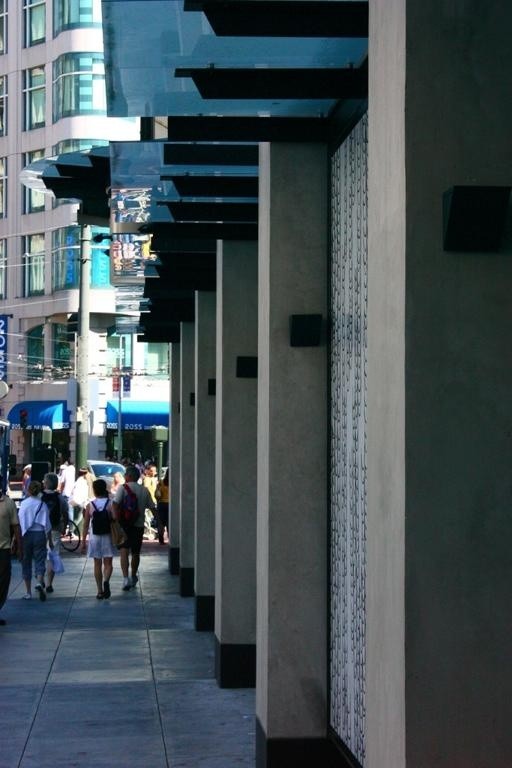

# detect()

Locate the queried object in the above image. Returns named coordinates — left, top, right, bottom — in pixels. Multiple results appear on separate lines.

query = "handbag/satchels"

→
left=154, top=479, right=163, bottom=501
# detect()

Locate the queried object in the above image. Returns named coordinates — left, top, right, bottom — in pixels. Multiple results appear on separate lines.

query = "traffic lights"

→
left=19, top=408, right=28, bottom=429
left=38, top=424, right=53, bottom=449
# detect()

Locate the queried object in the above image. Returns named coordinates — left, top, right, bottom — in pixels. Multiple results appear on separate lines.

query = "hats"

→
left=22, top=463, right=32, bottom=472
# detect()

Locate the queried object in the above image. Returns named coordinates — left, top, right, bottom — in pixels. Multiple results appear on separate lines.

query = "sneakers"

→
left=159, top=542, right=168, bottom=548
left=21, top=592, right=32, bottom=601
left=103, top=579, right=112, bottom=600
left=95, top=592, right=103, bottom=600
left=121, top=574, right=138, bottom=591
left=1, top=616, right=7, bottom=627
left=32, top=582, right=54, bottom=603
left=81, top=545, right=87, bottom=556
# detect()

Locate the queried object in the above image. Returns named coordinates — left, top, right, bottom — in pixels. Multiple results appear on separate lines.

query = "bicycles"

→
left=59, top=519, right=81, bottom=552
left=142, top=503, right=159, bottom=540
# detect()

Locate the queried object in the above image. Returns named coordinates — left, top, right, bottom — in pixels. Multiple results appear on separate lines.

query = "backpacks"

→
left=120, top=483, right=140, bottom=528
left=90, top=498, right=113, bottom=536
left=40, top=490, right=61, bottom=528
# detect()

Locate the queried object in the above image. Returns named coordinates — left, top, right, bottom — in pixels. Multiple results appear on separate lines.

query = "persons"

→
left=0, top=476, right=23, bottom=624
left=18, top=457, right=169, bottom=602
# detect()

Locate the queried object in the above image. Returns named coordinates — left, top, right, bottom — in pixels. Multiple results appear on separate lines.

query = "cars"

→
left=7, top=481, right=25, bottom=508
left=87, top=460, right=127, bottom=487
